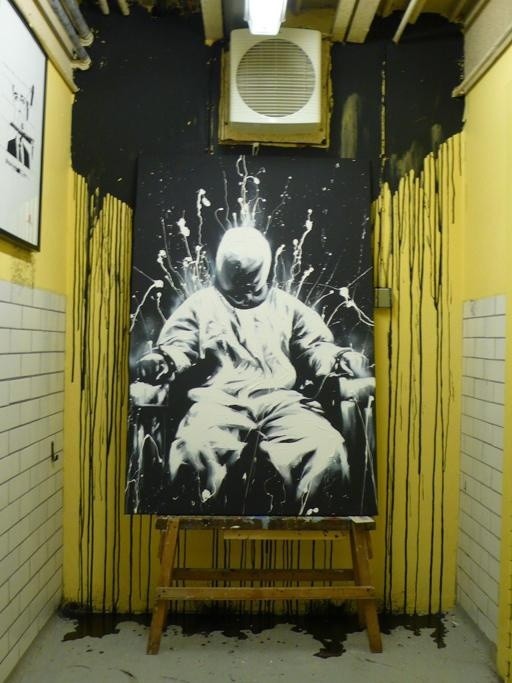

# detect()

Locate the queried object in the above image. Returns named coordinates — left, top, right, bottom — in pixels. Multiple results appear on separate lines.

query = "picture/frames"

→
left=0, top=0, right=49, bottom=250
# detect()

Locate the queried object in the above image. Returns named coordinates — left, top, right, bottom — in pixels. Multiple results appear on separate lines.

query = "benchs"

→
left=143, top=515, right=386, bottom=656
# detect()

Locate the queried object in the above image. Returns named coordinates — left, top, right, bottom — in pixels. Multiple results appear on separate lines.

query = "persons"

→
left=6, top=67, right=35, bottom=169
left=132, top=223, right=367, bottom=514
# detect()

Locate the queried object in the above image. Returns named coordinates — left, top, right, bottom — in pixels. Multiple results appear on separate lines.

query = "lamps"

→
left=240, top=0, right=292, bottom=38
